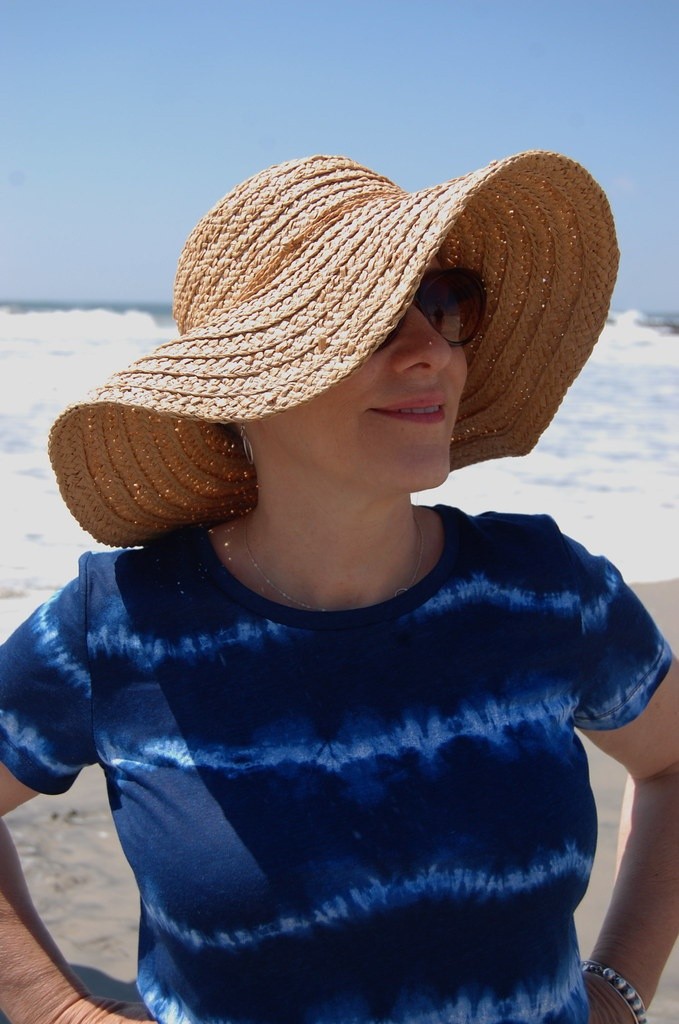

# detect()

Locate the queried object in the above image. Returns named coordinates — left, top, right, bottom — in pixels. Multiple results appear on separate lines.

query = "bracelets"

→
left=582, top=959, right=649, bottom=1024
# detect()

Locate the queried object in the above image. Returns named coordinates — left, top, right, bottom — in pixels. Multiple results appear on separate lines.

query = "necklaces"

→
left=244, top=507, right=424, bottom=612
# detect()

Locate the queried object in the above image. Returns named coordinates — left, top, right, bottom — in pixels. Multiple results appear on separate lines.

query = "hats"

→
left=44, top=149, right=621, bottom=550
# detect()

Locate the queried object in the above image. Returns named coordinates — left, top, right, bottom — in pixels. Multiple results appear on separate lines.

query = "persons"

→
left=0, top=151, right=679, bottom=1024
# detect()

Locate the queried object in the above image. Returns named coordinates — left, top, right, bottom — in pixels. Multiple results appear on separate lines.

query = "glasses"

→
left=374, top=266, right=487, bottom=346
